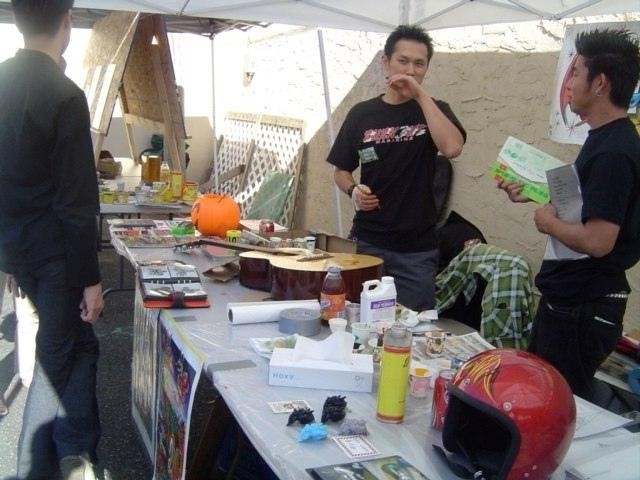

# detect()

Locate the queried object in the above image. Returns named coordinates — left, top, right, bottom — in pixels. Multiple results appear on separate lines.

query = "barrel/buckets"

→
left=360, top=275, right=396, bottom=327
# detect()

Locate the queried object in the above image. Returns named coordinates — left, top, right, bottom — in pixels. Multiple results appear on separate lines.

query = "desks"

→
left=110, top=215, right=640, bottom=480
left=95, top=167, right=212, bottom=295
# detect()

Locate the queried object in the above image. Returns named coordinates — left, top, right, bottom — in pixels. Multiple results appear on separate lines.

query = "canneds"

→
left=259, top=219, right=274, bottom=237
left=432, top=370, right=457, bottom=431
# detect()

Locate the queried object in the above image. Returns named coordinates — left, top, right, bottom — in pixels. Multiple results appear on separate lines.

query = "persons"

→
left=0, top=0, right=111, bottom=480
left=334, top=24, right=465, bottom=312
left=496, top=29, right=640, bottom=394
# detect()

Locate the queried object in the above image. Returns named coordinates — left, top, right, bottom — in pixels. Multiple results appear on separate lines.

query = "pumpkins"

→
left=191, top=195, right=240, bottom=239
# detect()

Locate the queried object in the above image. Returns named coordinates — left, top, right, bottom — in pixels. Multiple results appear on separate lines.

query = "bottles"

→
left=160, top=161, right=197, bottom=200
left=377, top=323, right=411, bottom=424
left=270, top=235, right=317, bottom=251
left=320, top=265, right=347, bottom=326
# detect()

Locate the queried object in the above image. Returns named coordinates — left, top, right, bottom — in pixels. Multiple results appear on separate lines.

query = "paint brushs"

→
left=347, top=176, right=381, bottom=210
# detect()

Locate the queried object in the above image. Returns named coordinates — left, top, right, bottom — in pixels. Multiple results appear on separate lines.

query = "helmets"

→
left=435, top=348, right=576, bottom=479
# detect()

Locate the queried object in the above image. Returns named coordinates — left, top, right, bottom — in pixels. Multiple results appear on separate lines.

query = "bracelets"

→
left=345, top=184, right=358, bottom=199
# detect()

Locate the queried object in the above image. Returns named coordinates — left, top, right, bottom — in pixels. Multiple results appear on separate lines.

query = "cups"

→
left=226, top=229, right=242, bottom=253
left=328, top=316, right=349, bottom=333
left=425, top=331, right=448, bottom=360
left=350, top=322, right=372, bottom=343
left=411, top=367, right=433, bottom=401
left=348, top=301, right=361, bottom=322
left=94, top=180, right=164, bottom=205
left=366, top=337, right=383, bottom=364
